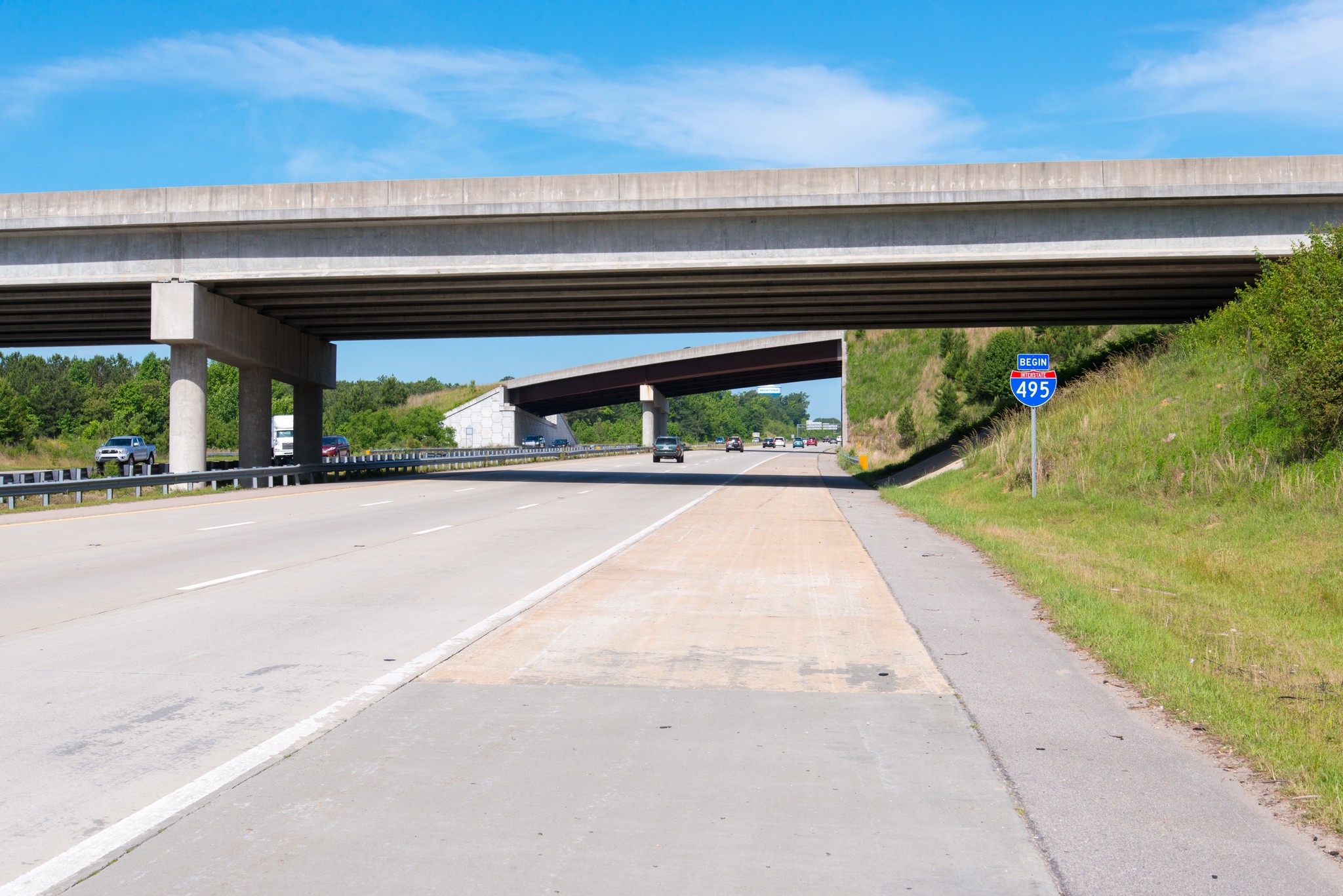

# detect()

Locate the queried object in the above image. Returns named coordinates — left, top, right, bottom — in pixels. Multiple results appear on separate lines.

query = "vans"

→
left=837, top=436, right=842, bottom=442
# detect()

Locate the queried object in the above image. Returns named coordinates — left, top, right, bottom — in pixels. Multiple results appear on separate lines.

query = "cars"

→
left=830, top=438, right=837, bottom=444
left=806, top=437, right=818, bottom=446
left=715, top=437, right=725, bottom=444
left=793, top=437, right=803, bottom=442
left=552, top=439, right=571, bottom=448
left=763, top=438, right=776, bottom=448
left=752, top=438, right=759, bottom=442
left=802, top=438, right=808, bottom=444
left=793, top=439, right=805, bottom=448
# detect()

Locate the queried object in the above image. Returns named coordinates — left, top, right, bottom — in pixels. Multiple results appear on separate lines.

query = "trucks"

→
left=271, top=414, right=293, bottom=459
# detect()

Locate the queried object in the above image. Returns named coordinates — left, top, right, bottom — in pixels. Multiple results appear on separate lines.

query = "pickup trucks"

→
left=95, top=436, right=156, bottom=465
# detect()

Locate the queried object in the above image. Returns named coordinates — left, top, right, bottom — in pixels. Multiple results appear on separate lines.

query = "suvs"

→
left=726, top=436, right=744, bottom=452
left=522, top=435, right=545, bottom=450
left=822, top=436, right=831, bottom=442
left=652, top=436, right=686, bottom=463
left=322, top=435, right=350, bottom=458
left=774, top=437, right=786, bottom=447
